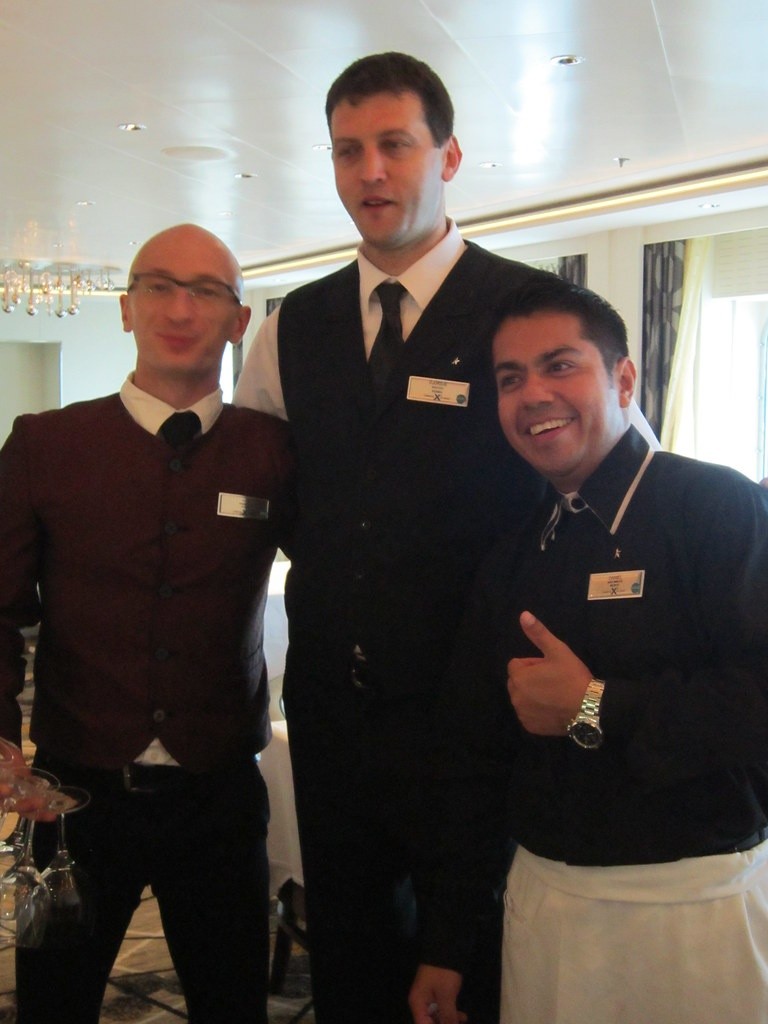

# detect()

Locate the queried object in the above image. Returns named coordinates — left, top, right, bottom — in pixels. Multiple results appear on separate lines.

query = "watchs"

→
left=566, top=677, right=606, bottom=749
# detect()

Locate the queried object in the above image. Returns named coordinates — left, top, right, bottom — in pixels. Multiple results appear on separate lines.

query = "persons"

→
left=0, top=224, right=300, bottom=1024
left=231, top=50, right=660, bottom=1024
left=404, top=274, right=768, bottom=1024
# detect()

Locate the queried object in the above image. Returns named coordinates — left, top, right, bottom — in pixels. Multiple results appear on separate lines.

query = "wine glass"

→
left=0, top=738, right=88, bottom=952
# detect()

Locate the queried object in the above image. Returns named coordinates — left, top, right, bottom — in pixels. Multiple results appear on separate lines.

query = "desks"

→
left=258, top=675, right=314, bottom=995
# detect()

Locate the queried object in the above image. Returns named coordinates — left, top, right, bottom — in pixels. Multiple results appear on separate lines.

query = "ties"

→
left=159, top=410, right=202, bottom=449
left=368, top=281, right=407, bottom=408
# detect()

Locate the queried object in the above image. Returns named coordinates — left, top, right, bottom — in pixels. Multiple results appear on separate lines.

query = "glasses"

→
left=127, top=272, right=243, bottom=307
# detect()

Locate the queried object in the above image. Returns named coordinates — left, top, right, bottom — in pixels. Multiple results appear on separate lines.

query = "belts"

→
left=37, top=745, right=261, bottom=795
left=691, top=825, right=768, bottom=856
